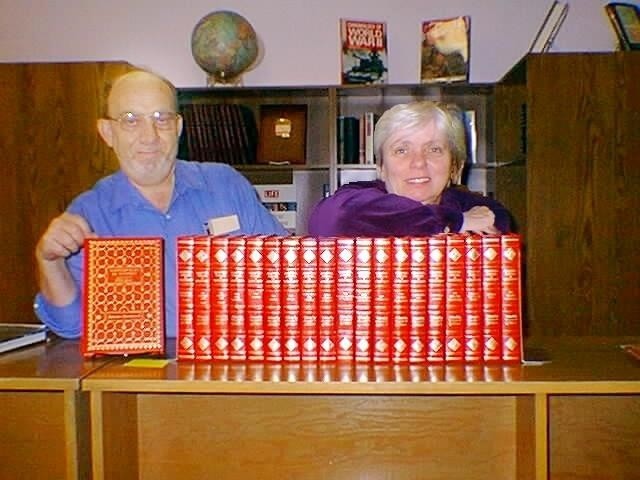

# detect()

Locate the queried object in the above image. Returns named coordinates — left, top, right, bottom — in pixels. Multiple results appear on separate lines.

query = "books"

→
left=0, top=320, right=49, bottom=354
left=176, top=100, right=257, bottom=165
left=346, top=117, right=359, bottom=164
left=461, top=109, right=479, bottom=165
left=78, top=234, right=167, bottom=360
left=174, top=231, right=524, bottom=366
left=338, top=115, right=346, bottom=164
left=258, top=102, right=309, bottom=163
left=417, top=15, right=473, bottom=83
left=337, top=16, right=389, bottom=86
left=529, top=1, right=571, bottom=54
left=358, top=116, right=365, bottom=165
left=604, top=2, right=640, bottom=52
left=364, top=110, right=375, bottom=165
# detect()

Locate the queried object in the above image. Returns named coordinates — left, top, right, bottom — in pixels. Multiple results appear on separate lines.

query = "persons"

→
left=310, top=100, right=530, bottom=345
left=31, top=67, right=296, bottom=341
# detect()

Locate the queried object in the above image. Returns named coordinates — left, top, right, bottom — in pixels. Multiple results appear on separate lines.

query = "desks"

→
left=80, top=333, right=638, bottom=480
left=0, top=333, right=129, bottom=480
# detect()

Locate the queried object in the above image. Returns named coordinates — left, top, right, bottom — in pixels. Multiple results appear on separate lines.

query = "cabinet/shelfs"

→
left=172, top=82, right=498, bottom=234
left=501, top=52, right=640, bottom=333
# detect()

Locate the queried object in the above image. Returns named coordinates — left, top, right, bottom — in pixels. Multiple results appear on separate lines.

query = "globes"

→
left=191, top=10, right=265, bottom=88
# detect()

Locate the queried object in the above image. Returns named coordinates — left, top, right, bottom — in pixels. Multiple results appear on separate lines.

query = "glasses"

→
left=104, top=110, right=179, bottom=133
